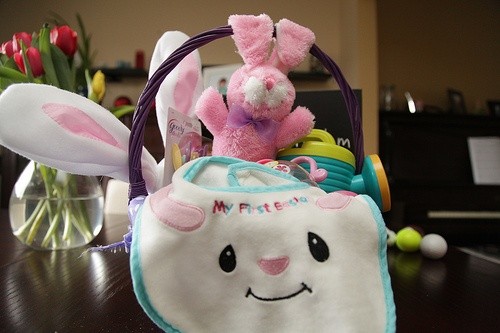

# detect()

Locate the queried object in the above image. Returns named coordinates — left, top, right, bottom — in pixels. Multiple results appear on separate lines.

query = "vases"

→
left=9, top=160, right=104, bottom=251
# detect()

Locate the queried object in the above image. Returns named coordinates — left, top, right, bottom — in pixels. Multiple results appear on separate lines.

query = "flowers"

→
left=0, top=12, right=134, bottom=248
